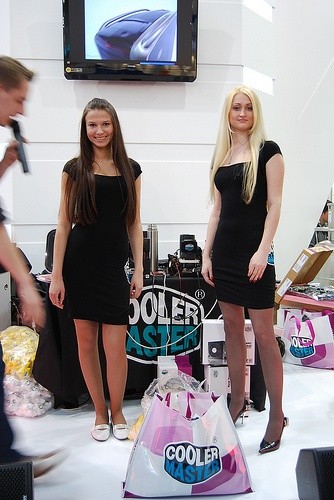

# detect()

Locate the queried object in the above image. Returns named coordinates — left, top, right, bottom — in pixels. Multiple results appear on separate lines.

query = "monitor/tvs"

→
left=61, top=0, right=198, bottom=83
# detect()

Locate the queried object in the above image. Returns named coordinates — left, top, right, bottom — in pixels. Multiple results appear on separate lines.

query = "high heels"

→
left=229, top=396, right=248, bottom=426
left=258, top=412, right=288, bottom=453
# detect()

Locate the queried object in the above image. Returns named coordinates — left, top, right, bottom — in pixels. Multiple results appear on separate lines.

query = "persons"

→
left=201, top=85, right=288, bottom=453
left=0, top=55, right=63, bottom=481
left=309, top=200, right=333, bottom=248
left=49, top=97, right=143, bottom=441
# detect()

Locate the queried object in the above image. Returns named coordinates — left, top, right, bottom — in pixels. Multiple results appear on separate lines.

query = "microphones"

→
left=10, top=119, right=28, bottom=173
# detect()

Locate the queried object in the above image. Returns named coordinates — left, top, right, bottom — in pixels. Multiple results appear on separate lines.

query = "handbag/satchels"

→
left=281, top=308, right=334, bottom=371
left=122, top=390, right=253, bottom=499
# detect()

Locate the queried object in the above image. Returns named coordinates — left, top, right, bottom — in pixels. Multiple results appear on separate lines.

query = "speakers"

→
left=0, top=461, right=34, bottom=500
left=296, top=446, right=334, bottom=500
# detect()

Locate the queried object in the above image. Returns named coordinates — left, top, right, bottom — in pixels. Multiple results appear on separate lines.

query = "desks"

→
left=32, top=267, right=265, bottom=413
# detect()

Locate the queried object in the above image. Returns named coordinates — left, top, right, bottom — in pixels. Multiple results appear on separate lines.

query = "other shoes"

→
left=109, top=413, right=130, bottom=439
left=91, top=412, right=111, bottom=442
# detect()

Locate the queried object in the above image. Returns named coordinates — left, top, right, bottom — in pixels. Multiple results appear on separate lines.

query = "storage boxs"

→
left=273, top=245, right=334, bottom=338
left=200, top=318, right=256, bottom=365
left=203, top=364, right=250, bottom=418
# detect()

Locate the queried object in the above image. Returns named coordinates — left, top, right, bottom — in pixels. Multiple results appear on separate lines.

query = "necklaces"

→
left=228, top=139, right=249, bottom=165
